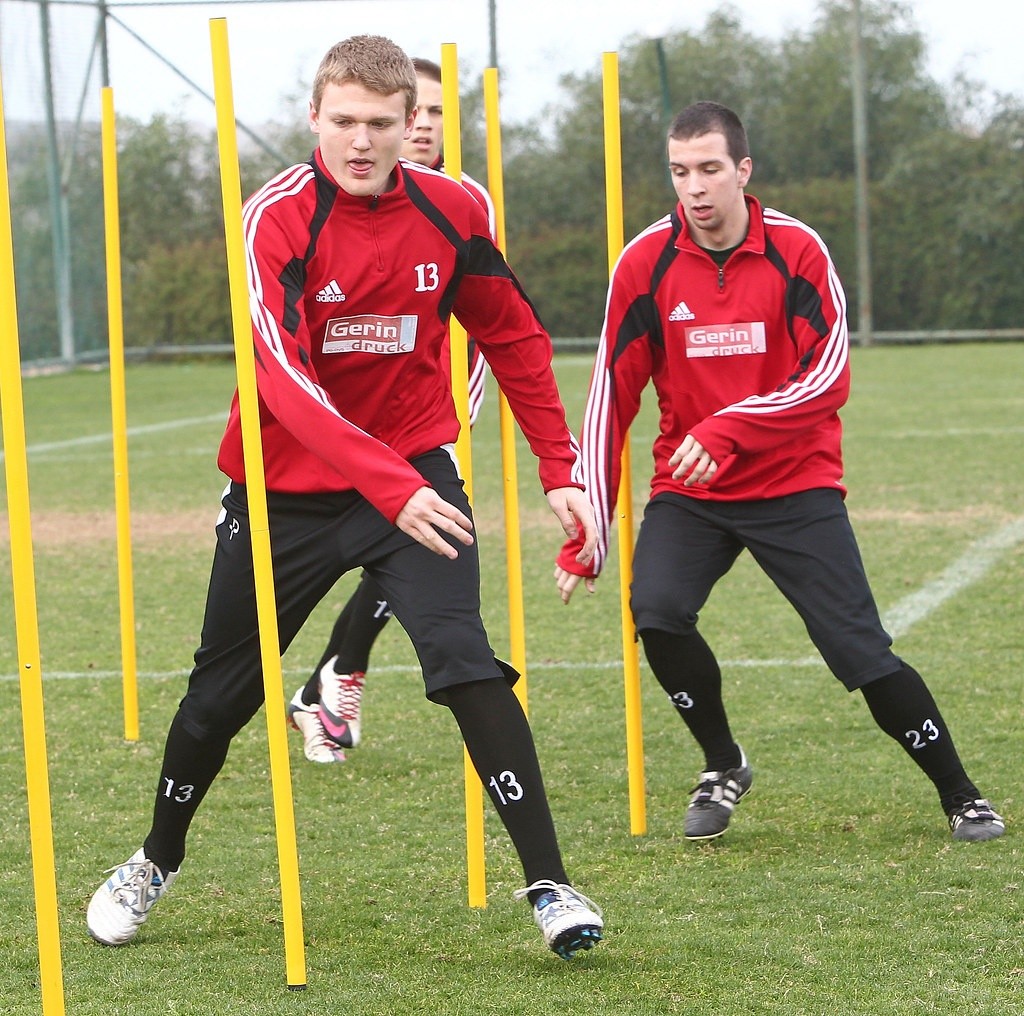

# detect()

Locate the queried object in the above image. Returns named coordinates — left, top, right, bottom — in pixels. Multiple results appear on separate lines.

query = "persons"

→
left=288, top=55, right=498, bottom=765
left=549, top=96, right=1009, bottom=847
left=80, top=35, right=608, bottom=963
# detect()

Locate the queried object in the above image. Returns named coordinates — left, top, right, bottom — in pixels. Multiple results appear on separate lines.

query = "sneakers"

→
left=948, top=798, right=1005, bottom=841
left=317, top=656, right=366, bottom=748
left=684, top=743, right=753, bottom=840
left=86, top=846, right=181, bottom=946
left=512, top=879, right=604, bottom=960
left=286, top=685, right=348, bottom=764
